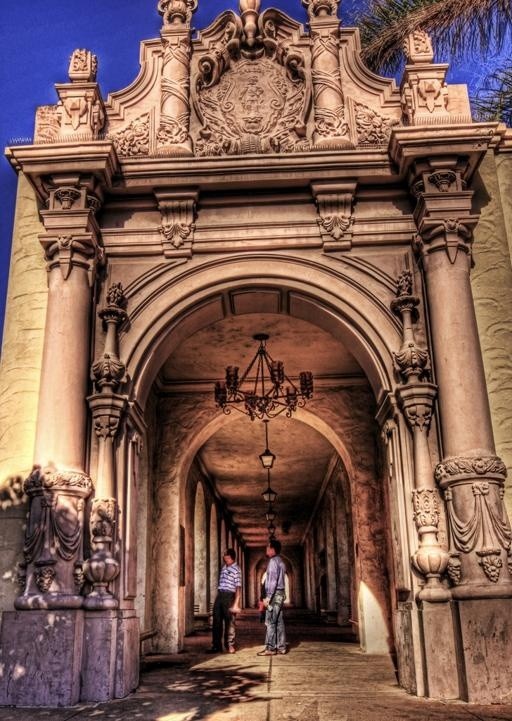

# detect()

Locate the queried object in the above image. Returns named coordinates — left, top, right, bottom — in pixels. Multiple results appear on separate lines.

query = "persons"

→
left=257, top=596, right=266, bottom=623
left=205, top=549, right=240, bottom=654
left=256, top=539, right=288, bottom=656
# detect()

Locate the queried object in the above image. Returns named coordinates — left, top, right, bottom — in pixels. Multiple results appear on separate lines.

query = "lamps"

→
left=257, top=419, right=279, bottom=523
left=212, top=333, right=314, bottom=422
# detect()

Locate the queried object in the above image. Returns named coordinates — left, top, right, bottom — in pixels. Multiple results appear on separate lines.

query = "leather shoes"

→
left=227, top=645, right=235, bottom=654
left=257, top=646, right=287, bottom=655
left=206, top=646, right=222, bottom=654
